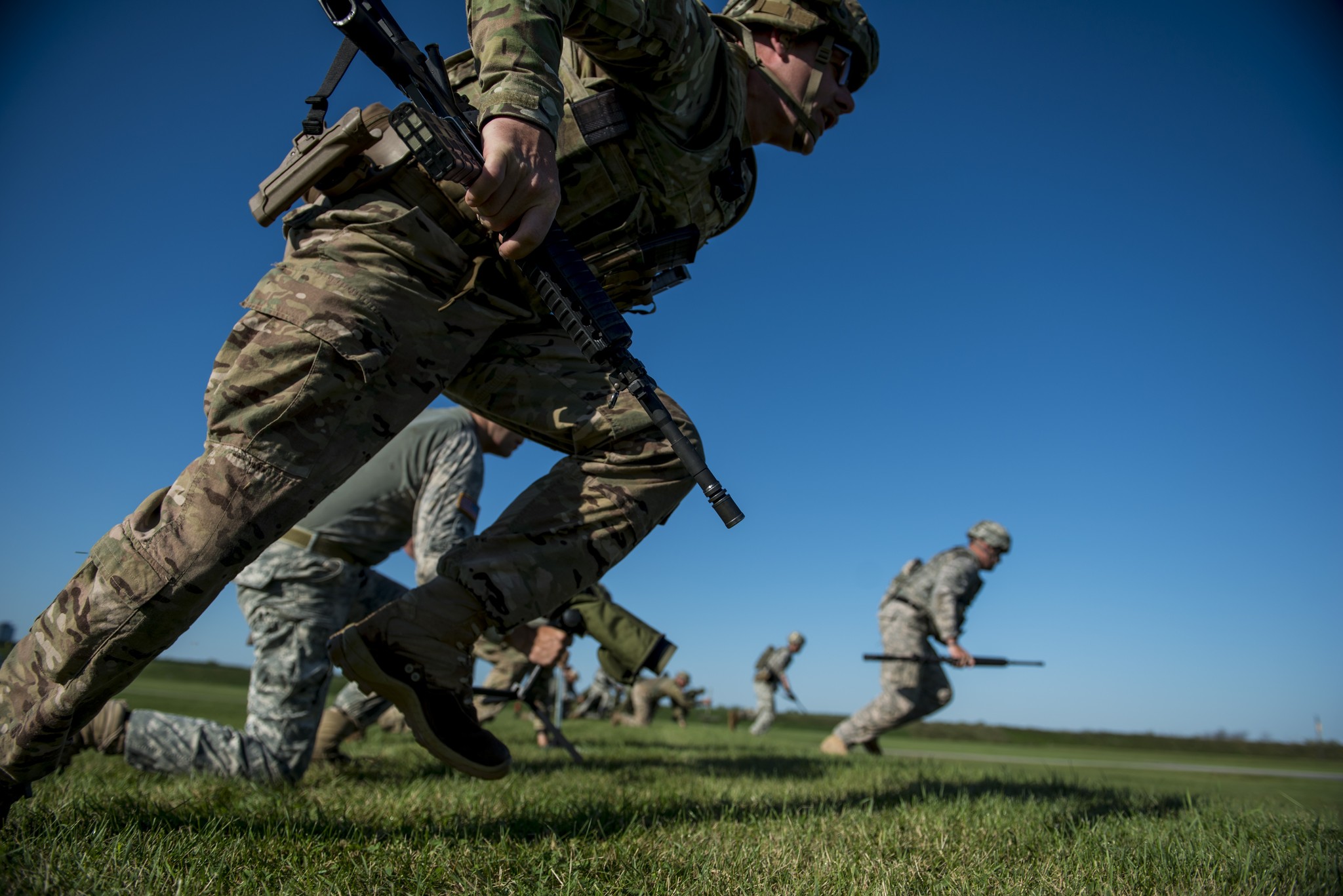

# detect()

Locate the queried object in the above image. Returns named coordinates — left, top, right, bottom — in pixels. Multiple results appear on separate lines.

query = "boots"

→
left=312, top=706, right=351, bottom=761
left=327, top=572, right=511, bottom=780
left=55, top=697, right=129, bottom=778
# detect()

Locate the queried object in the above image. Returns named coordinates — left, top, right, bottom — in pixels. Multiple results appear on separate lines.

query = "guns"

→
left=860, top=653, right=1048, bottom=668
left=470, top=606, right=595, bottom=773
left=298, top=0, right=752, bottom=533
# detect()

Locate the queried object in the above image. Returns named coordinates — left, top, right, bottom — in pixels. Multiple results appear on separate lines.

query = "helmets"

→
left=721, top=0, right=880, bottom=94
left=967, top=521, right=1010, bottom=552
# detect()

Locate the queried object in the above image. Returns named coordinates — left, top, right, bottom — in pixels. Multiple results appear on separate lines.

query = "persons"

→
left=67, top=400, right=567, bottom=786
left=728, top=628, right=805, bottom=737
left=819, top=517, right=1010, bottom=758
left=1, top=0, right=879, bottom=812
left=552, top=651, right=711, bottom=729
left=378, top=629, right=526, bottom=733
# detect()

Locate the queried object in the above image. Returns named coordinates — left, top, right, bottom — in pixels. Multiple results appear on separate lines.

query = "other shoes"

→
left=819, top=734, right=851, bottom=756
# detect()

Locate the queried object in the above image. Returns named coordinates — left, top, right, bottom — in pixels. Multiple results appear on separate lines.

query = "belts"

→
left=391, top=168, right=489, bottom=247
left=278, top=522, right=372, bottom=570
left=893, top=594, right=922, bottom=612
